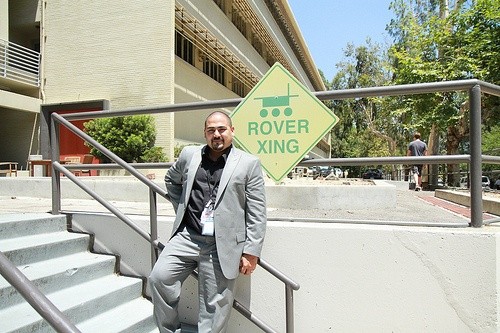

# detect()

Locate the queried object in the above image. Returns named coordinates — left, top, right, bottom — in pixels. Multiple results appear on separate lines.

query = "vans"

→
left=460, top=176, right=490, bottom=190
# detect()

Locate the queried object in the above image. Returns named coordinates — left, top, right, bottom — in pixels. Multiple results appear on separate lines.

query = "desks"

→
left=27, top=160, right=71, bottom=177
left=0, top=162, right=18, bottom=177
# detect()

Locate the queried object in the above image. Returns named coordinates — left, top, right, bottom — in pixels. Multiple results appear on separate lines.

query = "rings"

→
left=251, top=271, right=253, bottom=273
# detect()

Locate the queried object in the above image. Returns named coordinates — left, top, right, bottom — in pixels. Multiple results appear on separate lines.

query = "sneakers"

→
left=415, top=187, right=420, bottom=191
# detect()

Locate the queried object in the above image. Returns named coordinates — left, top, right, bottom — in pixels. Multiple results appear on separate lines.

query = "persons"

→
left=148, top=112, right=267, bottom=333
left=408, top=132, right=428, bottom=190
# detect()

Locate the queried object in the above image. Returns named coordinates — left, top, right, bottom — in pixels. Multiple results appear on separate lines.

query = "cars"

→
left=437, top=176, right=443, bottom=186
left=362, top=168, right=382, bottom=179
left=494, top=180, right=500, bottom=190
left=307, top=167, right=343, bottom=178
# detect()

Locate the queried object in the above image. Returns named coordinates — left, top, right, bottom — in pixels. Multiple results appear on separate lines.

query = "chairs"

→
left=63, top=157, right=81, bottom=177
left=74, top=154, right=94, bottom=176
